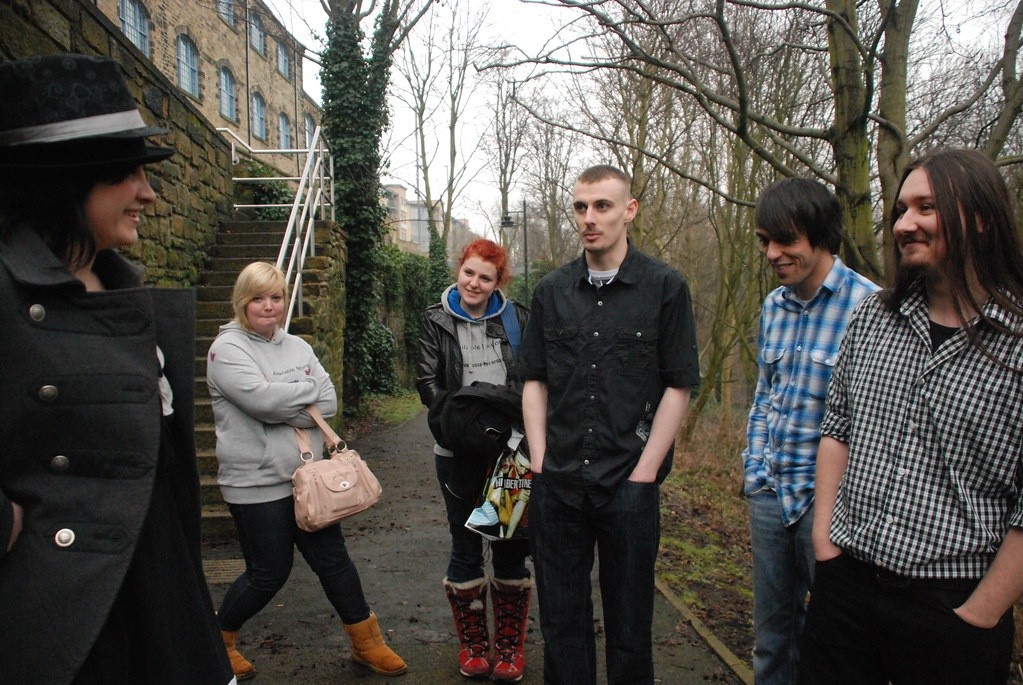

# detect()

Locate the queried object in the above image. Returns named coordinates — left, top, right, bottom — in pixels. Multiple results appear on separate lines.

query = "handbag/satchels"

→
left=464, top=445, right=531, bottom=542
left=291, top=404, right=383, bottom=533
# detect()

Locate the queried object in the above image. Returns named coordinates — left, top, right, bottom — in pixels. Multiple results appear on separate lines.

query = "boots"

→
left=491, top=576, right=532, bottom=683
left=443, top=573, right=489, bottom=677
left=343, top=612, right=408, bottom=676
left=220, top=629, right=255, bottom=681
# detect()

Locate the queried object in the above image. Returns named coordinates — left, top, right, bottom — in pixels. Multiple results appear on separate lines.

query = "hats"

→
left=0, top=54, right=176, bottom=178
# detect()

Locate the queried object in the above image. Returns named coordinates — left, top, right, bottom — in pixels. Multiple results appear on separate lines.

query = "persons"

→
left=518, top=165, right=700, bottom=685
left=413, top=239, right=532, bottom=682
left=208, top=262, right=407, bottom=679
left=0, top=57, right=234, bottom=685
left=794, top=147, right=1023, bottom=685
left=740, top=178, right=886, bottom=685
